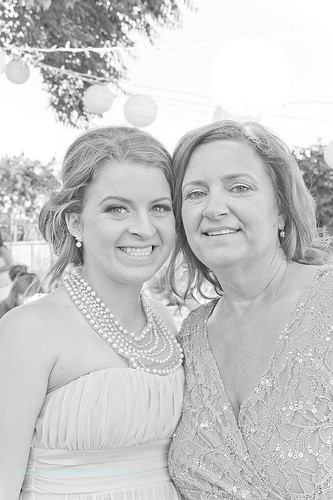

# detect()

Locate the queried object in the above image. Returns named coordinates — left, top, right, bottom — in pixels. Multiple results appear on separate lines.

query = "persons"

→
left=0, top=127, right=186, bottom=500
left=167, top=121, right=333, bottom=500
left=0, top=235, right=45, bottom=319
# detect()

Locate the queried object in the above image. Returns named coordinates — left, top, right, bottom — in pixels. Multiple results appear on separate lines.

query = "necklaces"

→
left=63, top=266, right=184, bottom=375
